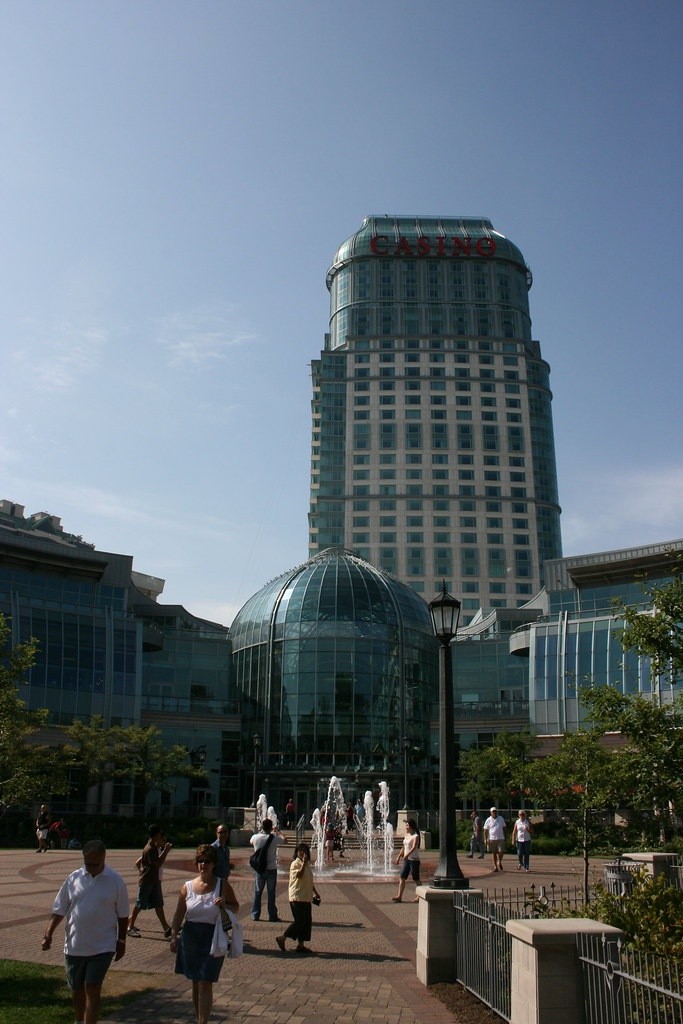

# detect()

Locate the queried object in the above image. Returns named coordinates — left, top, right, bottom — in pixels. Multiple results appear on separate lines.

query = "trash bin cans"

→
left=602, top=860, right=644, bottom=899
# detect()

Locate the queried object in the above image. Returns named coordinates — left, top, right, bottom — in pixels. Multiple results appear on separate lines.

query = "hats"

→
left=490, top=807, right=496, bottom=812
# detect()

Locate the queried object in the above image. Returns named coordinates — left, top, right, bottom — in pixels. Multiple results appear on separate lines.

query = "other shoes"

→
left=467, top=855, right=473, bottom=858
left=478, top=857, right=483, bottom=858
left=500, top=865, right=503, bottom=870
left=36, top=850, right=41, bottom=852
left=526, top=869, right=530, bottom=872
left=44, top=848, right=47, bottom=852
left=276, top=937, right=286, bottom=951
left=518, top=865, right=521, bottom=869
left=127, top=928, right=140, bottom=937
left=494, top=869, right=497, bottom=872
left=297, top=947, right=312, bottom=953
left=413, top=898, right=419, bottom=902
left=392, top=897, right=401, bottom=902
left=269, top=918, right=280, bottom=921
left=164, top=927, right=182, bottom=937
left=253, top=917, right=259, bottom=920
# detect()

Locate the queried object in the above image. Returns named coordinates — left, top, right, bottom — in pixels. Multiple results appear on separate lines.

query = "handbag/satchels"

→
left=250, top=834, right=274, bottom=874
left=220, top=878, right=232, bottom=931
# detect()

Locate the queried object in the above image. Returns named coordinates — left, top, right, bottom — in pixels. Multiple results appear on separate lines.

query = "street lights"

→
left=402, top=735, right=411, bottom=810
left=250, top=733, right=260, bottom=809
left=427, top=576, right=471, bottom=889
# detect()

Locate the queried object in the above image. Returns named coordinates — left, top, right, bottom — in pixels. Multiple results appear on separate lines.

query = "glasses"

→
left=85, top=860, right=102, bottom=866
left=197, top=859, right=211, bottom=864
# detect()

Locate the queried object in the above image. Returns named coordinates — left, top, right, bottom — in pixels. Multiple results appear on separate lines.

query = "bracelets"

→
left=119, top=939, right=125, bottom=943
left=44, top=937, right=52, bottom=941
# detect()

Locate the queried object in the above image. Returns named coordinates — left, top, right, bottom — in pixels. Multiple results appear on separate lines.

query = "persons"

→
left=286, top=798, right=297, bottom=829
left=127, top=824, right=184, bottom=938
left=48, top=818, right=68, bottom=848
left=325, top=822, right=335, bottom=860
left=320, top=799, right=326, bottom=829
left=483, top=807, right=506, bottom=872
left=465, top=810, right=485, bottom=860
left=40, top=838, right=130, bottom=1024
left=390, top=818, right=424, bottom=903
left=36, top=804, right=49, bottom=853
left=276, top=842, right=321, bottom=952
left=354, top=798, right=365, bottom=822
left=170, top=842, right=239, bottom=1024
left=512, top=811, right=533, bottom=874
left=249, top=819, right=288, bottom=922
left=344, top=801, right=354, bottom=832
left=210, top=824, right=236, bottom=880
